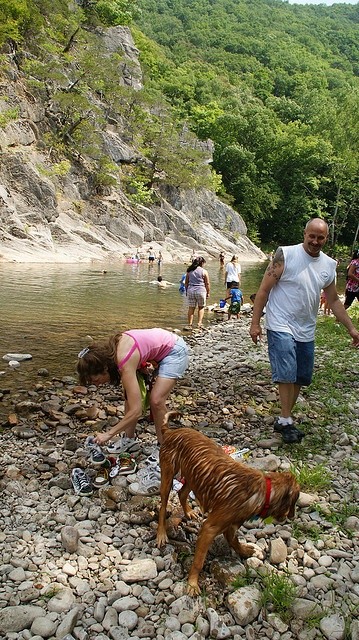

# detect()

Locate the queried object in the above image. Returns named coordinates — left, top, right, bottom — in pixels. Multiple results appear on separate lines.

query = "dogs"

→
left=152, top=429, right=302, bottom=598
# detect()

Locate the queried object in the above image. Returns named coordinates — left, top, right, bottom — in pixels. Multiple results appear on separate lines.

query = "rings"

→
left=96, top=441, right=99, bottom=444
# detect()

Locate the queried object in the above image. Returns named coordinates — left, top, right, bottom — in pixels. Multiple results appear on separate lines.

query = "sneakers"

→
left=129, top=472, right=161, bottom=496
left=84, top=436, right=106, bottom=464
left=106, top=433, right=142, bottom=453
left=94, top=459, right=112, bottom=488
left=282, top=424, right=302, bottom=444
left=118, top=452, right=137, bottom=475
left=145, top=446, right=161, bottom=466
left=70, top=468, right=93, bottom=497
left=273, top=420, right=305, bottom=437
left=126, top=465, right=161, bottom=483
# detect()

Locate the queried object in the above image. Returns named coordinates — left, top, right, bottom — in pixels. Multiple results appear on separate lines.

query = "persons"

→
left=136, top=251, right=141, bottom=263
left=157, top=250, right=163, bottom=266
left=343, top=249, right=359, bottom=310
left=224, top=255, right=242, bottom=303
left=184, top=256, right=211, bottom=329
left=250, top=294, right=256, bottom=304
left=190, top=249, right=199, bottom=263
left=146, top=246, right=156, bottom=263
left=76, top=327, right=190, bottom=447
left=219, top=252, right=224, bottom=265
left=178, top=268, right=189, bottom=294
left=334, top=258, right=340, bottom=285
left=131, top=254, right=135, bottom=259
left=224, top=281, right=244, bottom=320
left=158, top=276, right=163, bottom=282
left=249, top=217, right=359, bottom=444
